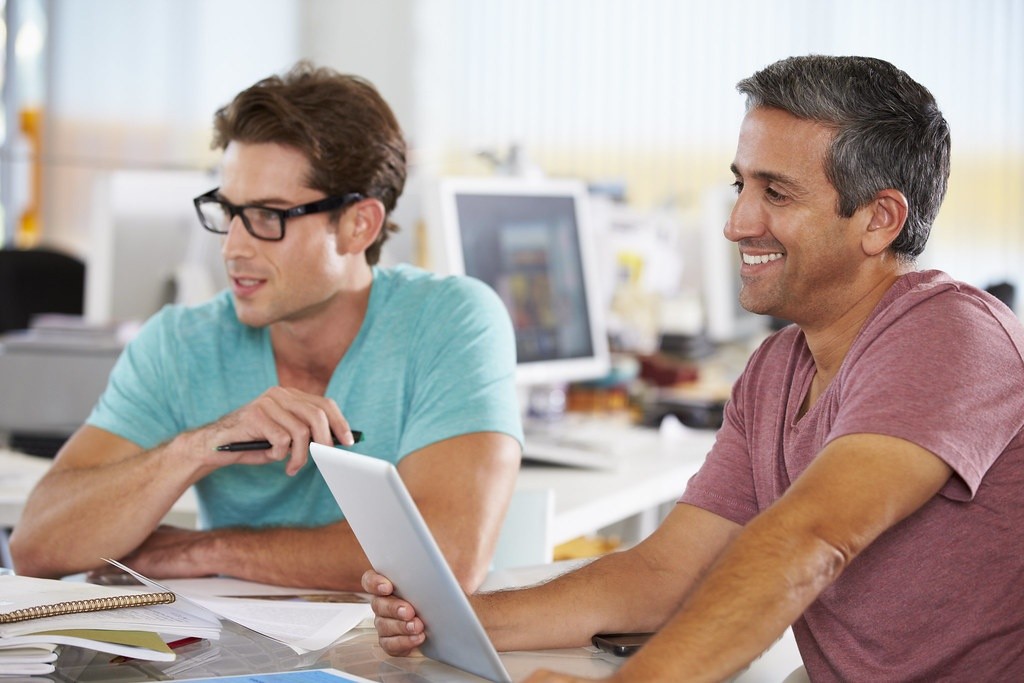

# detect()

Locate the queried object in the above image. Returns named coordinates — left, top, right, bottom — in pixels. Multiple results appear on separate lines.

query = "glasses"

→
left=193, top=187, right=400, bottom=242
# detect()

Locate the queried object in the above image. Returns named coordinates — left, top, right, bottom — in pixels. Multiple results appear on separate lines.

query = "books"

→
left=0, top=555, right=222, bottom=683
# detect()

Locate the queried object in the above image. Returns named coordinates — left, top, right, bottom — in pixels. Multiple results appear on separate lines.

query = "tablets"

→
left=309, top=442, right=512, bottom=683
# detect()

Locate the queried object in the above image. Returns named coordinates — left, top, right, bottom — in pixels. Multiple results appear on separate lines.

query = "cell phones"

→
left=592, top=632, right=657, bottom=657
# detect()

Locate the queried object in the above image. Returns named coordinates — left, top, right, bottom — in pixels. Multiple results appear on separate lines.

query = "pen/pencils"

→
left=211, top=430, right=366, bottom=453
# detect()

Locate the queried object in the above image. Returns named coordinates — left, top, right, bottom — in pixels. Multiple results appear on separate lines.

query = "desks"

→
left=310, top=426, right=809, bottom=683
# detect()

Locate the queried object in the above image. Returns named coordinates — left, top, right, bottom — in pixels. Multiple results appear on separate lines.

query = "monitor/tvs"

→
left=702, top=190, right=773, bottom=346
left=422, top=178, right=611, bottom=408
left=85, top=172, right=233, bottom=332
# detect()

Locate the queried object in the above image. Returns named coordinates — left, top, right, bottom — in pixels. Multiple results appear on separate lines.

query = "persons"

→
left=7, top=58, right=525, bottom=595
left=360, top=54, right=1024, bottom=683
